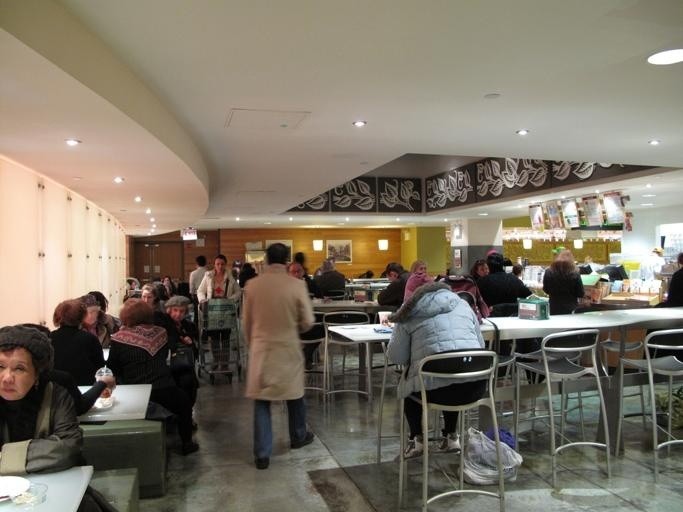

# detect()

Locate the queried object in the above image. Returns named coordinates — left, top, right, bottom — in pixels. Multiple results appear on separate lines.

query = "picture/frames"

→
left=325, top=239, right=353, bottom=263
left=264, top=239, right=294, bottom=264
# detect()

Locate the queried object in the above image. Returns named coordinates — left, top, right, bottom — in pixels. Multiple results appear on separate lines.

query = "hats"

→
left=323, top=260, right=334, bottom=271
left=0, top=325, right=55, bottom=378
left=164, top=295, right=191, bottom=307
left=403, top=270, right=435, bottom=303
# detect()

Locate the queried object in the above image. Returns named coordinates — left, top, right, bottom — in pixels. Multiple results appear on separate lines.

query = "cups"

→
left=377, top=311, right=391, bottom=327
left=93, top=367, right=115, bottom=399
left=354, top=289, right=365, bottom=302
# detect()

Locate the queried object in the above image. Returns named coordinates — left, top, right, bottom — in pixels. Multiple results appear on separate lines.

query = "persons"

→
left=665, top=253, right=683, bottom=306
left=243, top=241, right=316, bottom=469
left=584, top=256, right=593, bottom=267
left=24, top=277, right=200, bottom=455
left=376, top=251, right=532, bottom=381
left=543, top=249, right=586, bottom=314
left=189, top=256, right=209, bottom=344
left=289, top=262, right=327, bottom=370
left=196, top=254, right=243, bottom=373
left=385, top=280, right=486, bottom=462
left=0, top=324, right=117, bottom=511
left=230, top=252, right=346, bottom=318
left=639, top=247, right=666, bottom=281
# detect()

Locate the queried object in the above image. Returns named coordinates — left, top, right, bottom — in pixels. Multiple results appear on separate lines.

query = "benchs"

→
left=82, top=467, right=139, bottom=510
left=73, top=421, right=168, bottom=497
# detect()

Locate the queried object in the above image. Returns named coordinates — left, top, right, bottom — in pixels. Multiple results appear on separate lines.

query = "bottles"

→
left=516, top=257, right=542, bottom=282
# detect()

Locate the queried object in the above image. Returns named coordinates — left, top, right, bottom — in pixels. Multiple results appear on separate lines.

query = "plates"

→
left=0, top=474, right=30, bottom=502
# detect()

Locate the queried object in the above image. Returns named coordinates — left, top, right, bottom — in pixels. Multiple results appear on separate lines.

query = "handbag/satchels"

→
left=170, top=342, right=195, bottom=369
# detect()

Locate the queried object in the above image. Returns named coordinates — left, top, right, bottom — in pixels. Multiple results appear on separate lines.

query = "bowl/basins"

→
left=7, top=483, right=46, bottom=505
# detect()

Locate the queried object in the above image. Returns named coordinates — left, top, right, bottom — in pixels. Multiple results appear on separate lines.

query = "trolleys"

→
left=191, top=294, right=244, bottom=386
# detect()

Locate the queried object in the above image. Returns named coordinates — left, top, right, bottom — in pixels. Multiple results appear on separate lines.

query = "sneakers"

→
left=209, top=361, right=230, bottom=372
left=302, top=346, right=313, bottom=370
left=432, top=433, right=462, bottom=453
left=290, top=432, right=315, bottom=449
left=254, top=455, right=270, bottom=470
left=403, top=433, right=430, bottom=459
left=177, top=421, right=198, bottom=455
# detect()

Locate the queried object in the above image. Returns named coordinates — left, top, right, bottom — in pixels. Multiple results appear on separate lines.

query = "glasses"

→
left=288, top=268, right=305, bottom=275
